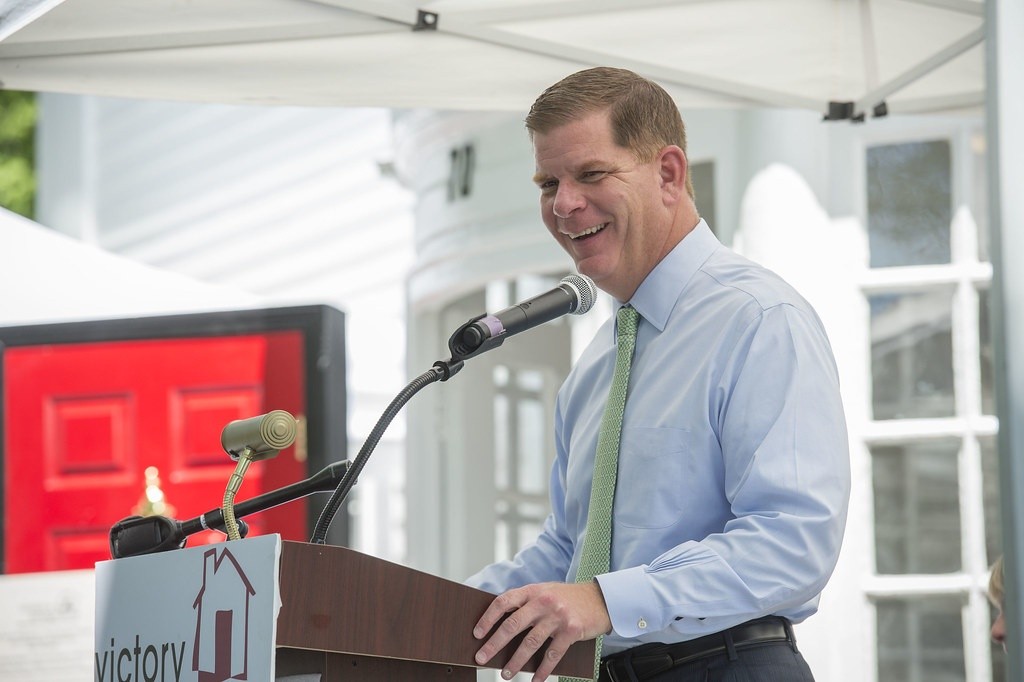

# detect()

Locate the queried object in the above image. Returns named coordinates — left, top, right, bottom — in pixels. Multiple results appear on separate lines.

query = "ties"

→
left=558, top=307, right=638, bottom=681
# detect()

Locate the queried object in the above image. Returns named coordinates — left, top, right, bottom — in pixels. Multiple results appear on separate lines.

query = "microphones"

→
left=464, top=272, right=597, bottom=347
left=109, top=460, right=358, bottom=560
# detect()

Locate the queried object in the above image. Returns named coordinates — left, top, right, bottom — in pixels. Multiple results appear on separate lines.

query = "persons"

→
left=984, top=555, right=1008, bottom=656
left=461, top=68, right=851, bottom=682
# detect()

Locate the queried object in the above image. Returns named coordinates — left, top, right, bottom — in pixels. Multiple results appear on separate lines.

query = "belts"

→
left=600, top=620, right=792, bottom=682
